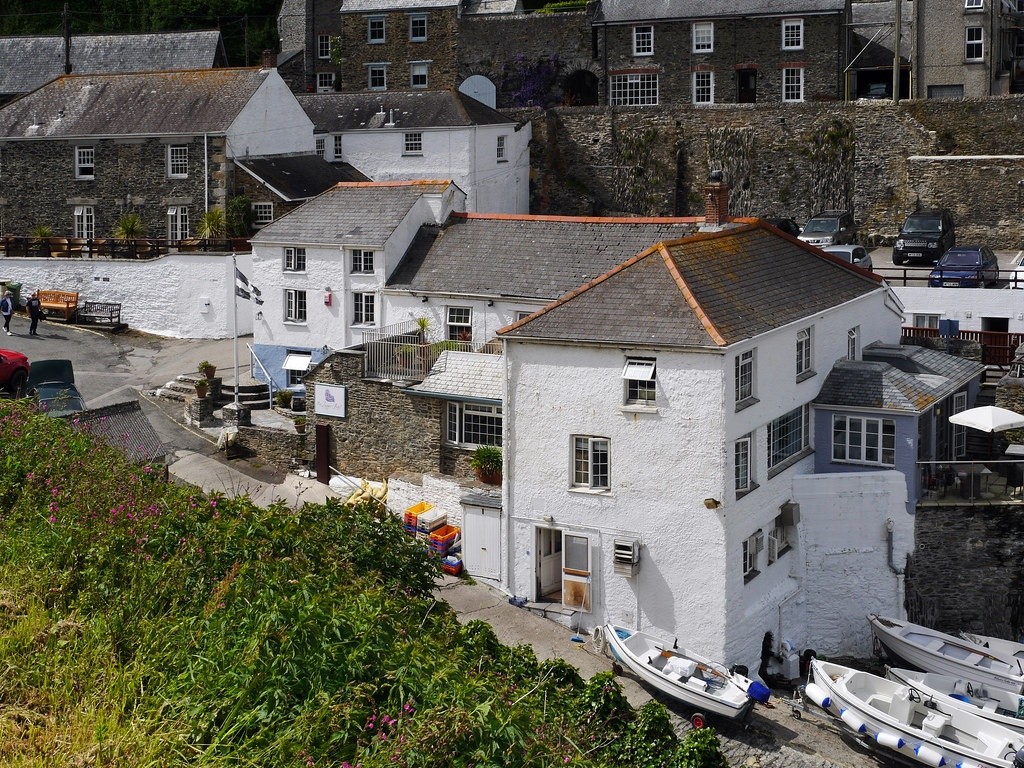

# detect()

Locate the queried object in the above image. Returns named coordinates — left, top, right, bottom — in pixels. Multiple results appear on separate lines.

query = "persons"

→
left=0, top=291, right=16, bottom=336
left=26, top=291, right=43, bottom=336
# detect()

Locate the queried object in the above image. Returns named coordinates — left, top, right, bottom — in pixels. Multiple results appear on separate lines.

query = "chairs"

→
left=50, top=237, right=152, bottom=258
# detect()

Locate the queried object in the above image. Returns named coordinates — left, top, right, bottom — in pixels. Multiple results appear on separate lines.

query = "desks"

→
left=1005, top=444, right=1024, bottom=456
left=951, top=464, right=992, bottom=499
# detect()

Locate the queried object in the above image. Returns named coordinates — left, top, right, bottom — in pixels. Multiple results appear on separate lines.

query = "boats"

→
left=866, top=614, right=1024, bottom=695
left=884, top=664, right=1024, bottom=736
left=602, top=623, right=771, bottom=724
left=810, top=655, right=1024, bottom=768
left=957, top=629, right=1024, bottom=659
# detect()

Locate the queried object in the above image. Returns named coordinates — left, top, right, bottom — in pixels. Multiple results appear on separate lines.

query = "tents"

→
left=949, top=406, right=1024, bottom=433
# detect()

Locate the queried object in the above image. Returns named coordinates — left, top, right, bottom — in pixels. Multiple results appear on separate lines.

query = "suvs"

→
left=797, top=210, right=856, bottom=249
left=892, top=207, right=956, bottom=266
left=929, top=245, right=999, bottom=289
left=763, top=218, right=801, bottom=238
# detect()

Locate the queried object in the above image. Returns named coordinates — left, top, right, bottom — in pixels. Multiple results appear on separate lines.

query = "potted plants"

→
left=395, top=317, right=431, bottom=367
left=197, top=361, right=217, bottom=379
left=293, top=416, right=307, bottom=433
left=194, top=380, right=209, bottom=398
left=469, top=444, right=502, bottom=485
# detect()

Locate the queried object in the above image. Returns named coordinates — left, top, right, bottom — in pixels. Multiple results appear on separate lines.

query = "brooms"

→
left=570, top=577, right=589, bottom=643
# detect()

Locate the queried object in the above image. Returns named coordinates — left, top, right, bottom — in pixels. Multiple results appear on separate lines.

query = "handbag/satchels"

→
left=38, top=311, right=46, bottom=321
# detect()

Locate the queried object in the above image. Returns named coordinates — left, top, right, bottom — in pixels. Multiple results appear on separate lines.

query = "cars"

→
left=0, top=348, right=29, bottom=393
left=857, top=80, right=892, bottom=102
left=16, top=360, right=86, bottom=419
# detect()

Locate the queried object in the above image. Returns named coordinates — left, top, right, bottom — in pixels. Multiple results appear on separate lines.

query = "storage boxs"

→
left=402, top=501, right=462, bottom=575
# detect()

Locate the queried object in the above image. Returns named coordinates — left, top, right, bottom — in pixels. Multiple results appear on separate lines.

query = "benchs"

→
left=37, top=289, right=79, bottom=319
left=74, top=301, right=121, bottom=324
left=667, top=671, right=683, bottom=680
left=964, top=653, right=984, bottom=664
left=1008, top=664, right=1024, bottom=677
left=926, top=640, right=946, bottom=651
left=982, top=699, right=1000, bottom=712
left=639, top=650, right=661, bottom=663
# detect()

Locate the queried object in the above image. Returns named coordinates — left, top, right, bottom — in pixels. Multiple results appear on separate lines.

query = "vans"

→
left=823, top=245, right=872, bottom=273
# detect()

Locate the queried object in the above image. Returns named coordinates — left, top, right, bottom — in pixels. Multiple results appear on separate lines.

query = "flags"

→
left=234, top=267, right=264, bottom=306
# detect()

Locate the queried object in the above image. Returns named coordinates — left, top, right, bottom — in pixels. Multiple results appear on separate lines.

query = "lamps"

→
left=704, top=499, right=721, bottom=509
left=934, top=402, right=941, bottom=415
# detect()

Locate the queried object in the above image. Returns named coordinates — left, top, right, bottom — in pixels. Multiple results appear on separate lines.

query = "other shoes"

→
left=2, top=327, right=7, bottom=331
left=29, top=332, right=32, bottom=336
left=33, top=332, right=38, bottom=335
left=7, top=331, right=12, bottom=336
